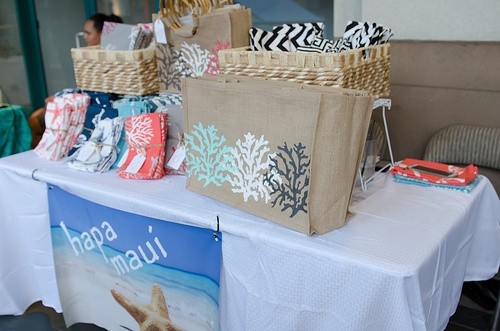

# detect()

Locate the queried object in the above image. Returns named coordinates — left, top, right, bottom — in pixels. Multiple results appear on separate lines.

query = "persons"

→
left=83, top=11, right=123, bottom=45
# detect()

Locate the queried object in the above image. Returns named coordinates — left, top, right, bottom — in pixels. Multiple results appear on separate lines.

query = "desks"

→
left=0, top=145, right=500, bottom=331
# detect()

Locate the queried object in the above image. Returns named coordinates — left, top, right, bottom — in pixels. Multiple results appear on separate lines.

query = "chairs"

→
left=423, top=124, right=500, bottom=170
